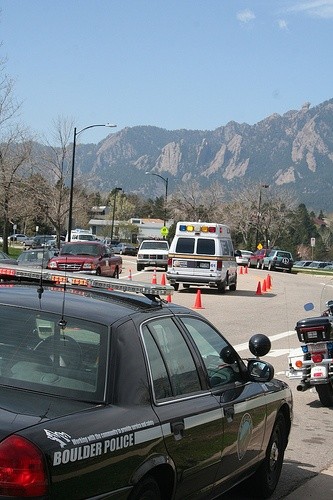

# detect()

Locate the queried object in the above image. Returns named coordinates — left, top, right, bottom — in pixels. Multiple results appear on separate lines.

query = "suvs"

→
left=247, top=249, right=271, bottom=269
left=262, top=250, right=294, bottom=273
left=166, top=221, right=240, bottom=293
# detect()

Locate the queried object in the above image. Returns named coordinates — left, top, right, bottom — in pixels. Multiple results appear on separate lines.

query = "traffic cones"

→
left=128, top=269, right=133, bottom=281
left=239, top=266, right=243, bottom=274
left=190, top=289, right=206, bottom=309
left=260, top=279, right=269, bottom=293
left=161, top=273, right=167, bottom=286
left=167, top=294, right=172, bottom=302
left=243, top=265, right=248, bottom=274
left=255, top=281, right=263, bottom=296
left=266, top=274, right=273, bottom=291
left=151, top=269, right=158, bottom=284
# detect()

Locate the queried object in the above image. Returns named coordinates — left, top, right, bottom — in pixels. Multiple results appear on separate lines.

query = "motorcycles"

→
left=286, top=279, right=333, bottom=406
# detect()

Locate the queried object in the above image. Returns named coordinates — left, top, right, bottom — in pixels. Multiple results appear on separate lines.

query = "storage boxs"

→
left=289, top=354, right=305, bottom=379
left=295, top=317, right=331, bottom=344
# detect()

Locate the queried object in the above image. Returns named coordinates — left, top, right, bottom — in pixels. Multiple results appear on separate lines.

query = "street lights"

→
left=144, top=171, right=169, bottom=240
left=111, top=187, right=122, bottom=243
left=256, top=185, right=269, bottom=252
left=68, top=122, right=118, bottom=241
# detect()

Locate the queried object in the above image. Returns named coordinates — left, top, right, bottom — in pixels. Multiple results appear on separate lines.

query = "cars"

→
left=0, top=262, right=294, bottom=500
left=0, top=231, right=140, bottom=271
left=136, top=240, right=170, bottom=272
left=47, top=240, right=123, bottom=280
left=235, top=250, right=254, bottom=265
left=292, top=260, right=333, bottom=272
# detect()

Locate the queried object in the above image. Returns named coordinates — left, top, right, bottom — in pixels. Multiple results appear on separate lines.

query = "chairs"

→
left=0, top=312, right=41, bottom=351
